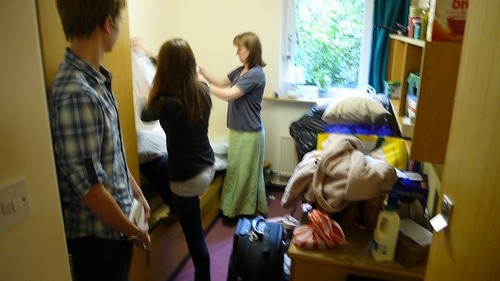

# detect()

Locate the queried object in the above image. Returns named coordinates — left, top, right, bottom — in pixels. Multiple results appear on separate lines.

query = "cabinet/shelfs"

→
left=382, top=31, right=465, bottom=165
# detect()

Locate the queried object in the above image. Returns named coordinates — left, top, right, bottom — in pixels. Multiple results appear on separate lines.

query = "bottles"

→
left=369, top=191, right=400, bottom=263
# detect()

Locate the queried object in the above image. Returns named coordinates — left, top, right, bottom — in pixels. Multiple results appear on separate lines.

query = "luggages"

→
left=227, top=216, right=284, bottom=281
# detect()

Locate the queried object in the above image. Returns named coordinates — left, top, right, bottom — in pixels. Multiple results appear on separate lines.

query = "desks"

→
left=289, top=158, right=429, bottom=281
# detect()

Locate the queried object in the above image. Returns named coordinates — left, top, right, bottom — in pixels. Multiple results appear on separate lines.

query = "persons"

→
left=197, top=32, right=269, bottom=217
left=47, top=0, right=151, bottom=281
left=131, top=38, right=216, bottom=281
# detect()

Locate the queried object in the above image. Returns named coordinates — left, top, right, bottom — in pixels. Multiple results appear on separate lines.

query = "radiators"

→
left=279, top=136, right=300, bottom=177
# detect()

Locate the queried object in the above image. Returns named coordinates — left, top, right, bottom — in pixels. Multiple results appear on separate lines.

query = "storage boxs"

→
left=426, top=0, right=469, bottom=43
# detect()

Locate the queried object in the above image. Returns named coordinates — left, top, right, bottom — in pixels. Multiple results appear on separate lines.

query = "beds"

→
left=140, top=149, right=226, bottom=281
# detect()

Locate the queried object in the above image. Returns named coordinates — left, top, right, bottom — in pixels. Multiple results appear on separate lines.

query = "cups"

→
left=408, top=16, right=421, bottom=39
